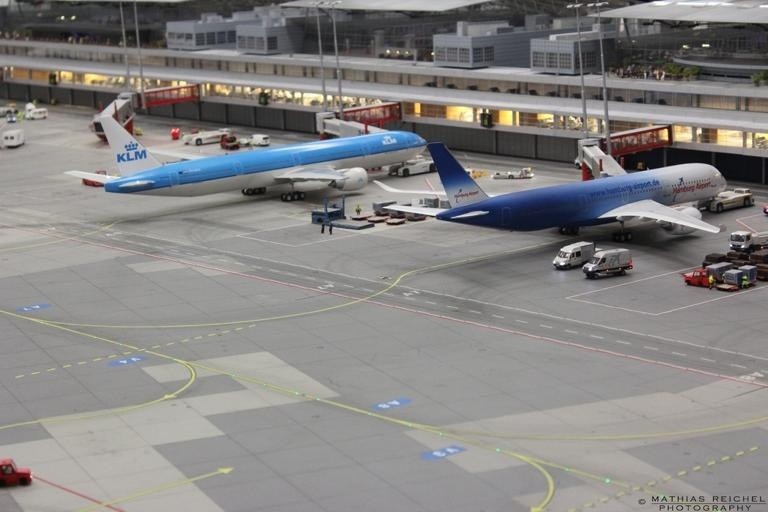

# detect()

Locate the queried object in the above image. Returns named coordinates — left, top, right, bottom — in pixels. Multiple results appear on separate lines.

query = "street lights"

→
left=566, top=0, right=611, bottom=154
left=309, top=0, right=344, bottom=120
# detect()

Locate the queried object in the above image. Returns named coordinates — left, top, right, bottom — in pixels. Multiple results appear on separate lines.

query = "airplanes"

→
left=64, top=113, right=727, bottom=241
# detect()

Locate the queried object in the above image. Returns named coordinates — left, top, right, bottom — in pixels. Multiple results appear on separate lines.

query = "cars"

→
left=0, top=457, right=33, bottom=488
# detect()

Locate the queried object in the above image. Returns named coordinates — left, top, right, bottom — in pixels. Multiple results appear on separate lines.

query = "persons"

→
left=356, top=205, right=361, bottom=215
left=329, top=224, right=333, bottom=234
left=321, top=223, right=325, bottom=232
left=741, top=274, right=749, bottom=288
left=708, top=273, right=714, bottom=288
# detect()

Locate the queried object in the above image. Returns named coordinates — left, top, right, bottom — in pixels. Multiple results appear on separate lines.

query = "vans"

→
left=552, top=240, right=633, bottom=278
left=25, top=102, right=48, bottom=120
left=3, top=129, right=25, bottom=148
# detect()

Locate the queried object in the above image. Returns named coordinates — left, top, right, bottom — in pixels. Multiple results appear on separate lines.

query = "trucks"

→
left=728, top=231, right=768, bottom=252
left=710, top=187, right=754, bottom=212
left=683, top=267, right=716, bottom=288
